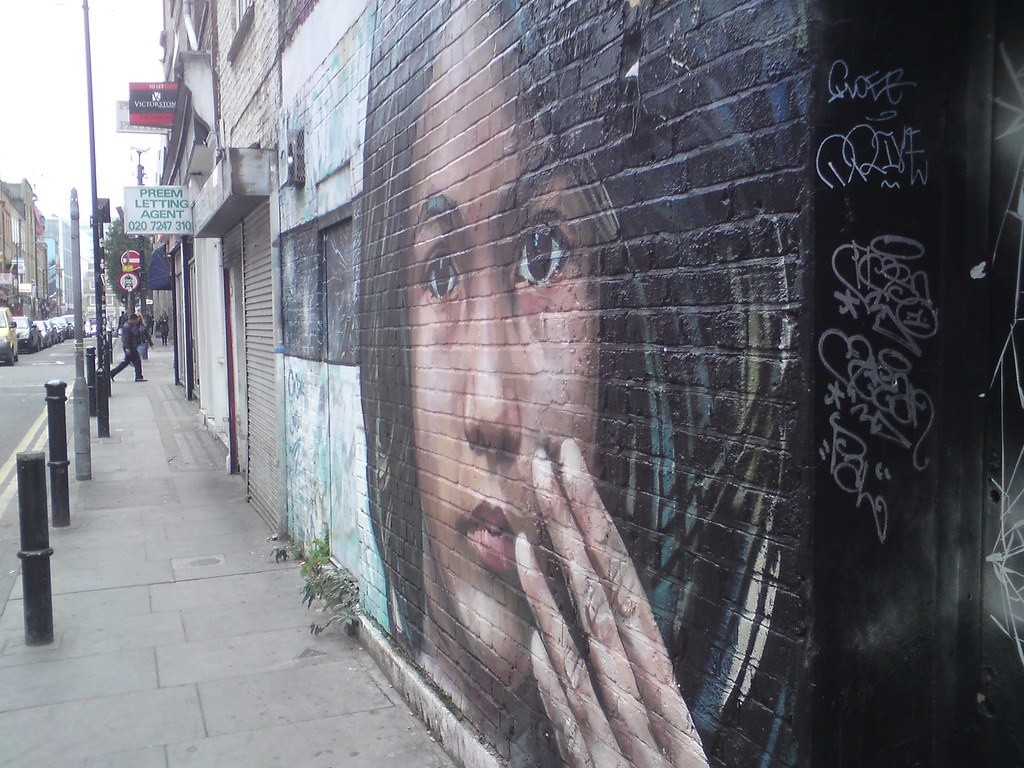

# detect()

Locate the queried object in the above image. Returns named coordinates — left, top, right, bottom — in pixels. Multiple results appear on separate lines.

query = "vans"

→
left=0, top=307, right=18, bottom=366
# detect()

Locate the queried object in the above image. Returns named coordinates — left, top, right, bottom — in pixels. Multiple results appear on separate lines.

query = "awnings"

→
left=148, top=243, right=172, bottom=290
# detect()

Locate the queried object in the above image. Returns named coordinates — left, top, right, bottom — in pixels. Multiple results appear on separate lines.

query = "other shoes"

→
left=110, top=373, right=114, bottom=381
left=135, top=378, right=147, bottom=382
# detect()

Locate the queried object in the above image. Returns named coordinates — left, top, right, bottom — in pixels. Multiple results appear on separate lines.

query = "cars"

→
left=13, top=315, right=93, bottom=354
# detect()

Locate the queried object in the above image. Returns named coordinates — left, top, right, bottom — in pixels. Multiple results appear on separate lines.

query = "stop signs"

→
left=121, top=251, right=140, bottom=271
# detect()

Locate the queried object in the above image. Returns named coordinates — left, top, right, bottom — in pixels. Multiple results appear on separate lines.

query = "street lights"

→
left=97, top=198, right=112, bottom=397
left=16, top=241, right=46, bottom=306
left=132, top=147, right=149, bottom=322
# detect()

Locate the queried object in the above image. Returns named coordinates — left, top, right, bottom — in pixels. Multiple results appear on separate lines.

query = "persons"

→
left=110, top=314, right=147, bottom=382
left=117, top=310, right=169, bottom=348
